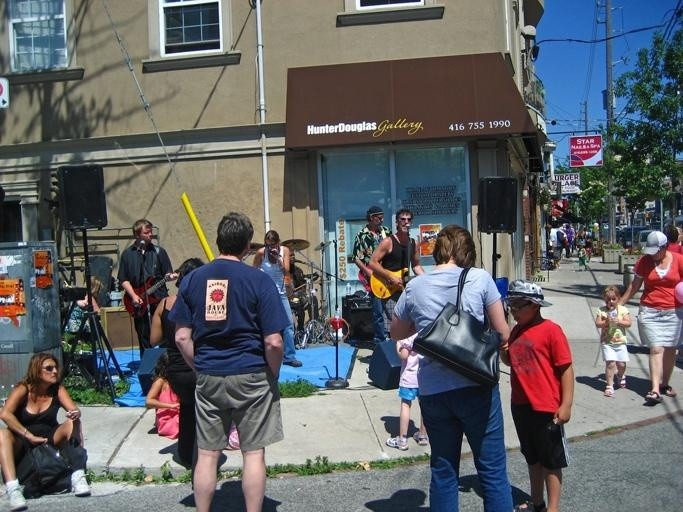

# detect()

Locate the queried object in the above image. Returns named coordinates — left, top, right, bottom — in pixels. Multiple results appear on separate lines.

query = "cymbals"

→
left=315, top=240, right=331, bottom=252
left=311, top=280, right=330, bottom=284
left=280, top=239, right=309, bottom=252
left=240, top=240, right=268, bottom=256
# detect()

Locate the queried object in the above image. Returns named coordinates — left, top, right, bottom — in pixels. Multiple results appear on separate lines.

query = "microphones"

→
left=137, top=240, right=147, bottom=257
left=270, top=249, right=282, bottom=258
left=406, top=224, right=410, bottom=237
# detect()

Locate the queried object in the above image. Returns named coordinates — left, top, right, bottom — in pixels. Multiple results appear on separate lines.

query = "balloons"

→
left=674, top=282, right=683, bottom=303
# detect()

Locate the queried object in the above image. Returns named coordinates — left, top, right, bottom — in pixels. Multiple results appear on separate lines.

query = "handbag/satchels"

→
left=412, top=265, right=501, bottom=388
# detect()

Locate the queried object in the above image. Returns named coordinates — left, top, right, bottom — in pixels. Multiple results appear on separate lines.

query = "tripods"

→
left=62, top=229, right=124, bottom=401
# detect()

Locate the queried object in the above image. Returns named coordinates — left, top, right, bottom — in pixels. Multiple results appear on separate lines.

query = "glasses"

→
left=507, top=301, right=532, bottom=311
left=264, top=243, right=277, bottom=248
left=373, top=215, right=383, bottom=220
left=42, top=365, right=57, bottom=371
left=400, top=218, right=412, bottom=220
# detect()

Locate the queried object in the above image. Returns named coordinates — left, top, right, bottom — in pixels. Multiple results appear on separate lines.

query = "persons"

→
left=500, top=279, right=574, bottom=512
left=116, top=219, right=173, bottom=361
left=0, top=353, right=90, bottom=511
left=353, top=205, right=392, bottom=343
left=253, top=230, right=303, bottom=368
left=65, top=276, right=104, bottom=333
left=168, top=212, right=291, bottom=512
left=559, top=224, right=593, bottom=259
left=386, top=332, right=427, bottom=449
left=595, top=284, right=631, bottom=398
left=146, top=352, right=181, bottom=440
left=663, top=225, right=683, bottom=254
left=676, top=226, right=683, bottom=245
left=369, top=208, right=426, bottom=339
left=288, top=247, right=319, bottom=330
left=149, top=257, right=205, bottom=467
left=620, top=231, right=683, bottom=402
left=389, top=225, right=512, bottom=512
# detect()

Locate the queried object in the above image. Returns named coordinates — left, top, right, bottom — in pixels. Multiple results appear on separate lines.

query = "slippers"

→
left=660, top=383, right=676, bottom=397
left=645, top=391, right=663, bottom=403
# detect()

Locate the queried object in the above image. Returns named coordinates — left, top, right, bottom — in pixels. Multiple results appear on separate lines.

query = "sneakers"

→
left=6, top=479, right=27, bottom=512
left=71, top=469, right=92, bottom=497
left=386, top=438, right=409, bottom=451
left=284, top=360, right=302, bottom=367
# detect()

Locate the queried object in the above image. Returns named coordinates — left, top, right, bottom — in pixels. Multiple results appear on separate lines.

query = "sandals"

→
left=515, top=501, right=546, bottom=512
left=604, top=386, right=615, bottom=397
left=399, top=446, right=402, bottom=447
left=414, top=431, right=428, bottom=446
left=616, top=374, right=626, bottom=389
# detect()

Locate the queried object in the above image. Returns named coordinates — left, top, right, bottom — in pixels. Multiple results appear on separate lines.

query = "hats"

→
left=641, top=231, right=667, bottom=255
left=577, top=242, right=585, bottom=246
left=366, top=205, right=384, bottom=221
left=502, top=279, right=553, bottom=306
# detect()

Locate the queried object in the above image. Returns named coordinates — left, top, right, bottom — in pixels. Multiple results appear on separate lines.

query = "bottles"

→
left=608, top=307, right=617, bottom=328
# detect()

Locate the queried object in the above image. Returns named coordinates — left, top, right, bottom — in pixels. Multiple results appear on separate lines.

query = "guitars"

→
left=369, top=266, right=419, bottom=299
left=122, top=271, right=180, bottom=319
left=358, top=231, right=442, bottom=292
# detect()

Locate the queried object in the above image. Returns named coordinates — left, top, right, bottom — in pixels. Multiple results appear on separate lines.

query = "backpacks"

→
left=16, top=439, right=87, bottom=499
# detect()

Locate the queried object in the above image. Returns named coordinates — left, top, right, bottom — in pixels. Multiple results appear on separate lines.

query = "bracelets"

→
left=23, top=427, right=27, bottom=439
left=65, top=411, right=78, bottom=422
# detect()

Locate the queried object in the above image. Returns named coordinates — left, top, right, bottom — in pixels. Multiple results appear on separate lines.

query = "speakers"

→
left=55, top=164, right=108, bottom=231
left=479, top=177, right=517, bottom=234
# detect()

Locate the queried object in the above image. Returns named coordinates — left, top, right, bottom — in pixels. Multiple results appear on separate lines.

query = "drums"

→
left=323, top=315, right=351, bottom=342
